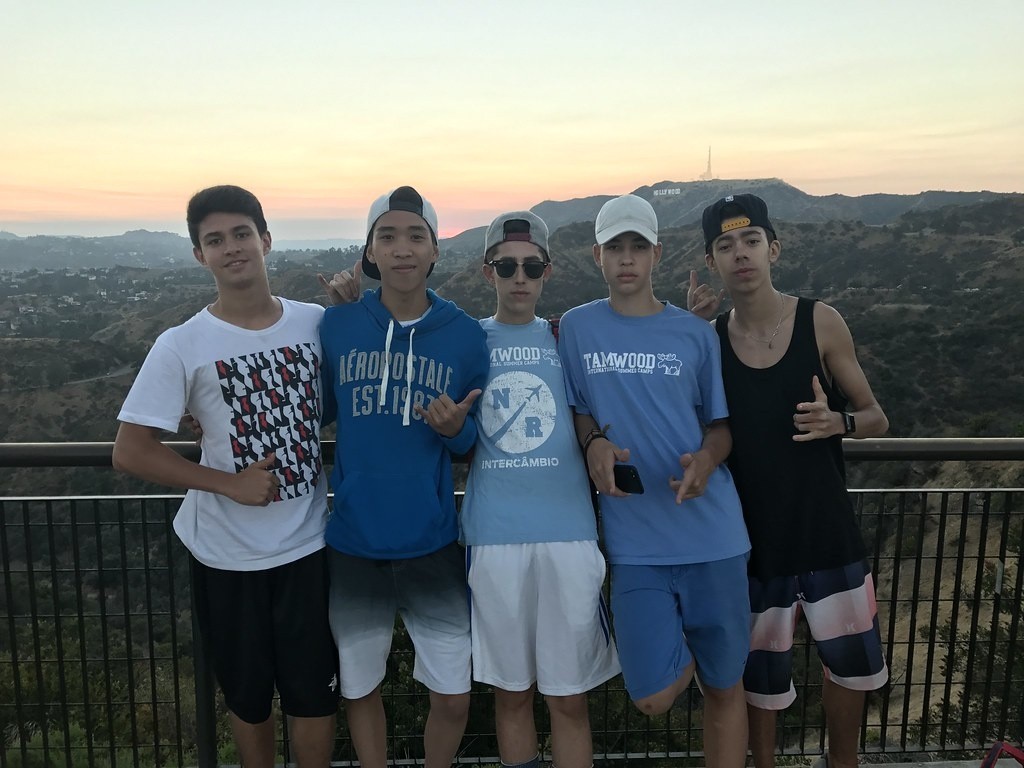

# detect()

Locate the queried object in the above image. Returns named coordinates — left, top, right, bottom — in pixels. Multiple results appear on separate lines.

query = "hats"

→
left=702, top=193, right=777, bottom=254
left=595, top=194, right=658, bottom=246
left=362, top=186, right=438, bottom=280
left=484, top=211, right=551, bottom=263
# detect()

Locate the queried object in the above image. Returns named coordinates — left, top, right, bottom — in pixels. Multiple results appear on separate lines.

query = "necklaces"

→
left=733, top=291, right=786, bottom=349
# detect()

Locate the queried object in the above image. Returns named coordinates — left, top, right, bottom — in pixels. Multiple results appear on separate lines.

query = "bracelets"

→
left=581, top=424, right=610, bottom=458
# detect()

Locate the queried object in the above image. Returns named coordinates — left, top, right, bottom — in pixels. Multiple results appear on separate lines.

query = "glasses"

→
left=489, top=259, right=549, bottom=279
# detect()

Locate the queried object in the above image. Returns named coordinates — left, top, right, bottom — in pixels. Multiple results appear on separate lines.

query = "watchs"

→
left=841, top=411, right=856, bottom=438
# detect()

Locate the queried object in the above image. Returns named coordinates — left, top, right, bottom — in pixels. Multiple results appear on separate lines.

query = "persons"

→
left=557, top=194, right=750, bottom=768
left=180, top=185, right=491, bottom=768
left=319, top=210, right=621, bottom=768
left=685, top=193, right=890, bottom=768
left=111, top=185, right=363, bottom=768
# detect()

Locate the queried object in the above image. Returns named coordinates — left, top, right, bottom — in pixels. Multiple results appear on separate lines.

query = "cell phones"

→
left=613, top=465, right=644, bottom=495
